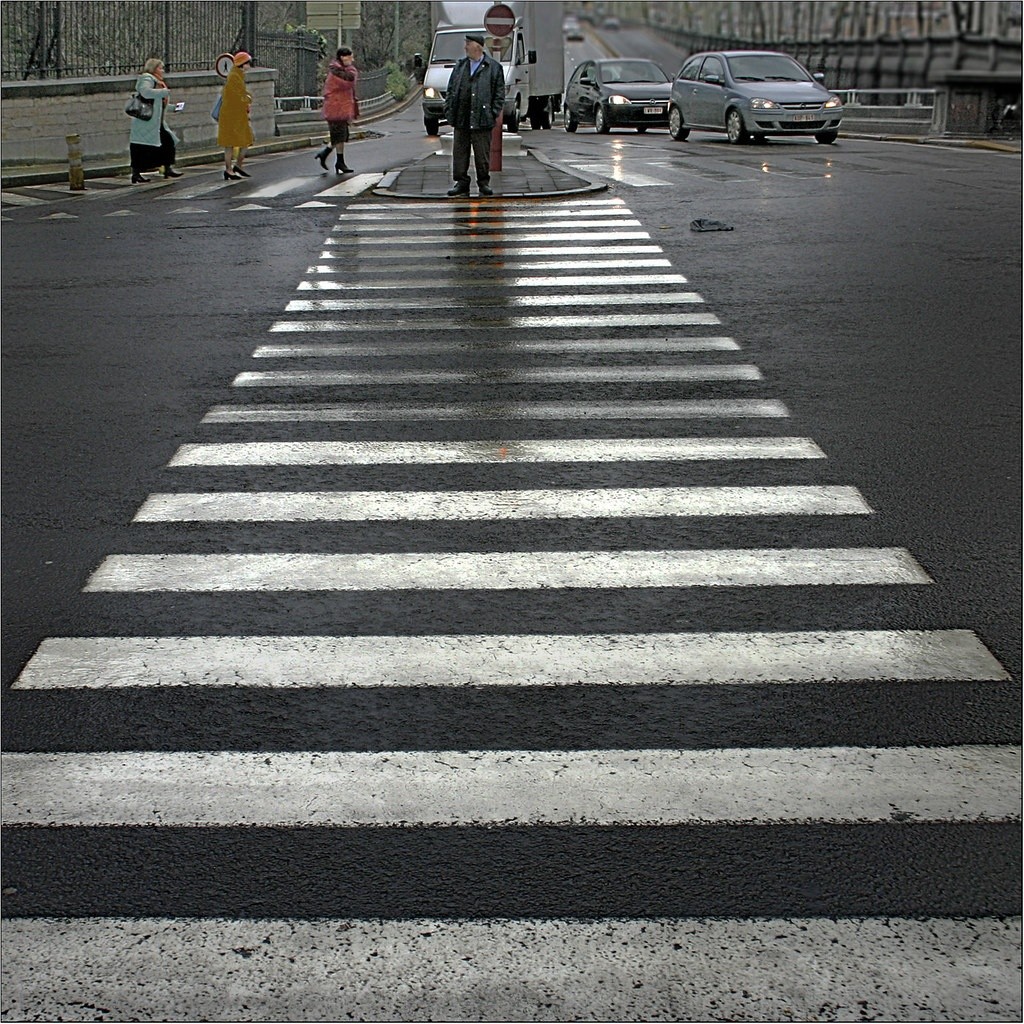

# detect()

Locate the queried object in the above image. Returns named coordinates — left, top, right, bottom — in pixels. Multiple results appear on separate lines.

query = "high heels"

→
left=224, top=171, right=242, bottom=180
left=163, top=168, right=183, bottom=179
left=335, top=161, right=354, bottom=173
left=315, top=152, right=329, bottom=170
left=233, top=165, right=251, bottom=177
left=132, top=173, right=151, bottom=183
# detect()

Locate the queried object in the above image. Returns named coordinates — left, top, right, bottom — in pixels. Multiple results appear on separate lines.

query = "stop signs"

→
left=486, top=4, right=516, bottom=37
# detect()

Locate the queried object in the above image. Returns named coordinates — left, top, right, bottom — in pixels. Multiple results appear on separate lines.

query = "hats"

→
left=464, top=35, right=484, bottom=47
left=234, top=52, right=252, bottom=67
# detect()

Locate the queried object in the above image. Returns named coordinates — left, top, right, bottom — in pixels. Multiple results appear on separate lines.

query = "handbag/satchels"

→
left=212, top=99, right=222, bottom=121
left=125, top=94, right=153, bottom=121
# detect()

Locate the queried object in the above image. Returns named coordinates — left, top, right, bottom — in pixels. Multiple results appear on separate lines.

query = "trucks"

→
left=414, top=1, right=566, bottom=136
left=563, top=57, right=673, bottom=135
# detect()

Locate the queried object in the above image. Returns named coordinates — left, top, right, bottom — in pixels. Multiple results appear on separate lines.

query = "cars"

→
left=602, top=16, right=622, bottom=31
left=666, top=49, right=845, bottom=145
left=561, top=15, right=585, bottom=42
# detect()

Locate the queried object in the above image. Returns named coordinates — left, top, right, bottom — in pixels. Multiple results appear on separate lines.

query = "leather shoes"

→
left=478, top=184, right=493, bottom=196
left=447, top=183, right=470, bottom=196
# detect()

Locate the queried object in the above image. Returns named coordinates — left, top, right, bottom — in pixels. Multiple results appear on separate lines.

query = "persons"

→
left=129, top=59, right=184, bottom=183
left=314, top=46, right=359, bottom=174
left=217, top=52, right=255, bottom=180
left=443, top=34, right=505, bottom=196
